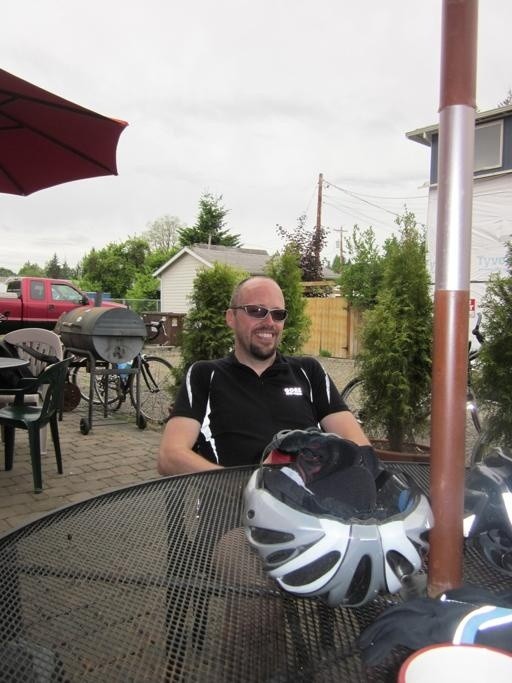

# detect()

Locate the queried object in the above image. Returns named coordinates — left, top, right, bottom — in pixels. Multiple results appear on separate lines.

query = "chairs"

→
left=0, top=328, right=76, bottom=493
left=163, top=474, right=335, bottom=683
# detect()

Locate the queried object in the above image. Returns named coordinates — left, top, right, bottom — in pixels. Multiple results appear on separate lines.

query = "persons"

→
left=154, top=272, right=390, bottom=683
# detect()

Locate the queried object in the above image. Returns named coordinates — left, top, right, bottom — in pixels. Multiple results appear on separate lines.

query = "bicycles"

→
left=69, top=353, right=128, bottom=407
left=89, top=319, right=183, bottom=423
left=335, top=310, right=497, bottom=436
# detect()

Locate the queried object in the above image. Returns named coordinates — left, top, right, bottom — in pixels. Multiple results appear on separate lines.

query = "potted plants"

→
left=354, top=291, right=433, bottom=463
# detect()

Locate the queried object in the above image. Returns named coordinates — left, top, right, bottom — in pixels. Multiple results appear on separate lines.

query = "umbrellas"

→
left=0, top=64, right=132, bottom=200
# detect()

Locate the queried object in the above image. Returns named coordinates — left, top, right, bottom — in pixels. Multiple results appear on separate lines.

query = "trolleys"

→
left=50, top=342, right=149, bottom=434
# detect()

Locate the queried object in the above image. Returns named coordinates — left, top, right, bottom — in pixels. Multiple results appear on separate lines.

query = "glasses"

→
left=231, top=302, right=290, bottom=323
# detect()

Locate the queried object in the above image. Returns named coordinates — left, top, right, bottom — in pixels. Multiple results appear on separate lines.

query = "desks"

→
left=0, top=460, right=512, bottom=682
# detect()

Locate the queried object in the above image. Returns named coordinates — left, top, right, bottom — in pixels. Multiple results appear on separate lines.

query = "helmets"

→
left=463, top=446, right=512, bottom=578
left=241, top=424, right=436, bottom=611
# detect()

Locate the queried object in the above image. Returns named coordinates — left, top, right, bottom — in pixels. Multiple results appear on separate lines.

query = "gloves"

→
left=355, top=596, right=512, bottom=671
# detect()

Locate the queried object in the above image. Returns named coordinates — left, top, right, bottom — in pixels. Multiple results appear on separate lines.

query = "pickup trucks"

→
left=0, top=273, right=125, bottom=334
